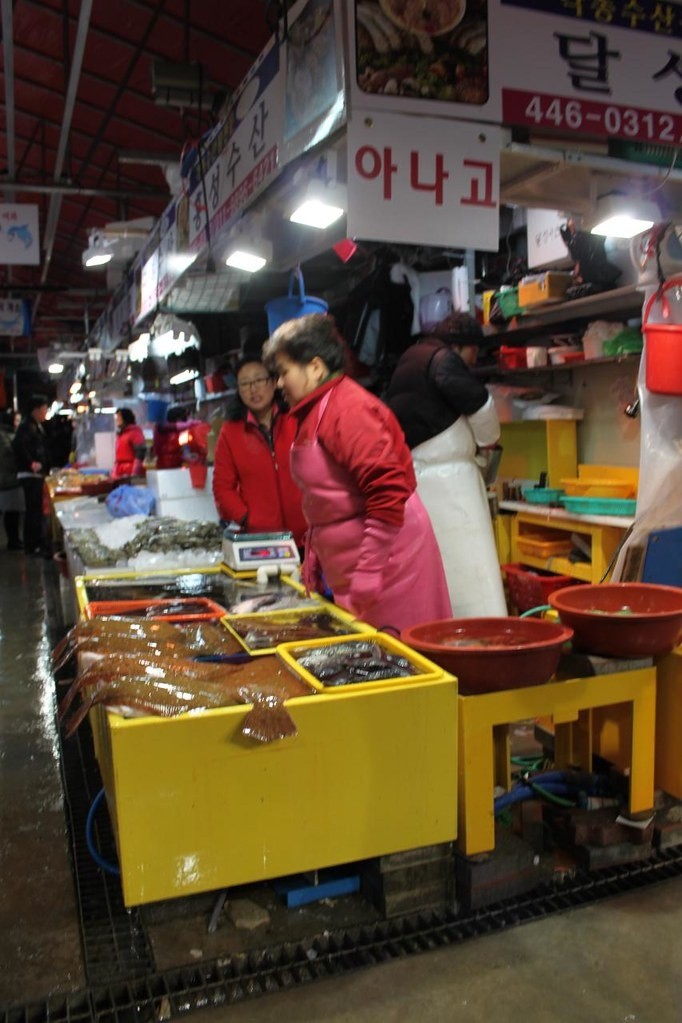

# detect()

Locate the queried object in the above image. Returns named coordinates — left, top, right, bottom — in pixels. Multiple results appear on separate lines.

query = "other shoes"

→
left=25, top=545, right=52, bottom=559
left=6, top=539, right=23, bottom=551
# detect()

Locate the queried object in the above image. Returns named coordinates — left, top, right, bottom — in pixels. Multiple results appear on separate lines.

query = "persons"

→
left=13, top=395, right=56, bottom=559
left=112, top=407, right=147, bottom=488
left=193, top=354, right=309, bottom=561
left=263, top=312, right=453, bottom=640
left=0, top=413, right=26, bottom=551
left=382, top=311, right=509, bottom=618
left=149, top=405, right=188, bottom=469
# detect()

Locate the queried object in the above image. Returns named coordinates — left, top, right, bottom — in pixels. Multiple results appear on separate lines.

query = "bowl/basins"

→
left=548, top=584, right=682, bottom=653
left=559, top=466, right=640, bottom=499
left=401, top=615, right=574, bottom=694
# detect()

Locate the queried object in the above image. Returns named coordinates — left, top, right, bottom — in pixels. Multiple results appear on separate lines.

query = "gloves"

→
left=130, top=457, right=143, bottom=477
left=348, top=517, right=399, bottom=615
left=300, top=547, right=321, bottom=592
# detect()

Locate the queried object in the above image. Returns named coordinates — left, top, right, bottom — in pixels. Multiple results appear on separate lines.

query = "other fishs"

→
left=58, top=653, right=243, bottom=719
left=66, top=676, right=246, bottom=738
left=126, top=517, right=223, bottom=555
left=231, top=660, right=298, bottom=743
left=49, top=615, right=231, bottom=676
left=234, top=620, right=333, bottom=651
left=297, top=643, right=410, bottom=686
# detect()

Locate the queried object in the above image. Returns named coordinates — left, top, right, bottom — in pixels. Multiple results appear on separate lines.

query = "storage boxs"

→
left=519, top=271, right=571, bottom=306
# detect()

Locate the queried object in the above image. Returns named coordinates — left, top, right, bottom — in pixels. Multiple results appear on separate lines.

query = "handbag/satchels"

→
left=105, top=485, right=154, bottom=517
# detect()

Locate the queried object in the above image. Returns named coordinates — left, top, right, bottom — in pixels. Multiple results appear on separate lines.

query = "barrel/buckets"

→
left=145, top=396, right=169, bottom=423
left=264, top=268, right=329, bottom=336
left=640, top=278, right=681, bottom=397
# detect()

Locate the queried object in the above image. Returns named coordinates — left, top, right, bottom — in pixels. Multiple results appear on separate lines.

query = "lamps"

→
left=591, top=195, right=660, bottom=240
left=50, top=156, right=350, bottom=385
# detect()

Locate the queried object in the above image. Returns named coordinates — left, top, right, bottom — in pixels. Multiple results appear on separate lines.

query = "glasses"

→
left=236, top=374, right=272, bottom=394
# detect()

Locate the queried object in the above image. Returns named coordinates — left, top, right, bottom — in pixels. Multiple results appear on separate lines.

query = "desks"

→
left=499, top=503, right=639, bottom=591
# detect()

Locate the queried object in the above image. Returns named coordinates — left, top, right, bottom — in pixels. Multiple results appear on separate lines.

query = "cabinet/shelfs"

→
left=489, top=280, right=644, bottom=377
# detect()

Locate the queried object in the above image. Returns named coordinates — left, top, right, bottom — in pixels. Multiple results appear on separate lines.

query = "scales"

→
left=224, top=531, right=300, bottom=571
